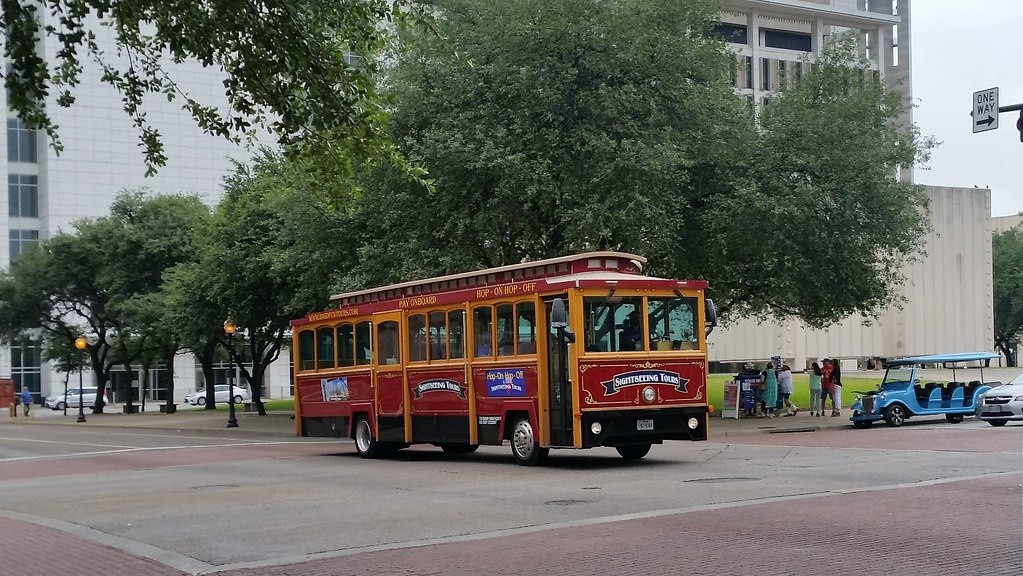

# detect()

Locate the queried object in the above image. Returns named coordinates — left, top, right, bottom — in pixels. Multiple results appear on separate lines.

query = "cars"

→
left=46, top=388, right=109, bottom=411
left=977, top=371, right=1023, bottom=426
left=184, top=385, right=248, bottom=406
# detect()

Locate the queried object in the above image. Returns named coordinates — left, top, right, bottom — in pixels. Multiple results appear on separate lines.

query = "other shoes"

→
left=761, top=412, right=766, bottom=418
left=822, top=411, right=825, bottom=416
left=752, top=413, right=757, bottom=416
left=783, top=413, right=793, bottom=417
left=810, top=412, right=813, bottom=416
left=746, top=413, right=753, bottom=416
left=816, top=413, right=820, bottom=417
left=832, top=411, right=835, bottom=414
left=792, top=407, right=800, bottom=416
left=831, top=412, right=840, bottom=417
left=26, top=412, right=28, bottom=416
left=769, top=416, right=773, bottom=419
left=766, top=413, right=769, bottom=417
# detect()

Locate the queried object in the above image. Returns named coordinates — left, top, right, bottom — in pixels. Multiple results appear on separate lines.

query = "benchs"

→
left=122, top=405, right=141, bottom=413
left=303, top=358, right=371, bottom=370
left=242, top=402, right=266, bottom=412
left=915, top=380, right=981, bottom=409
left=159, top=404, right=180, bottom=413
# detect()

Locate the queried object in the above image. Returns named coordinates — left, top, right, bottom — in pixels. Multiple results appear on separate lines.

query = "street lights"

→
left=75, top=335, right=89, bottom=422
left=223, top=314, right=241, bottom=428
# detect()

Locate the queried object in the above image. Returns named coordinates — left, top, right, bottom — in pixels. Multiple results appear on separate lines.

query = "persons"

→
left=740, top=362, right=762, bottom=416
left=14, top=392, right=20, bottom=417
left=759, top=362, right=779, bottom=418
left=776, top=364, right=798, bottom=417
left=804, top=362, right=822, bottom=417
left=820, top=358, right=835, bottom=416
left=829, top=359, right=842, bottom=417
left=22, top=387, right=33, bottom=417
left=478, top=332, right=490, bottom=357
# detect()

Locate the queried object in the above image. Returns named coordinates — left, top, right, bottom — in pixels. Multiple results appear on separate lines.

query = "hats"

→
left=821, top=358, right=829, bottom=362
left=626, top=311, right=639, bottom=319
left=767, top=363, right=773, bottom=369
left=24, top=387, right=27, bottom=390
left=744, top=362, right=754, bottom=369
left=781, top=361, right=792, bottom=370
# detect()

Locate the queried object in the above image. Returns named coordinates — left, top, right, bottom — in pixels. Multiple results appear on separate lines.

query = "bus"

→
left=287, top=252, right=717, bottom=466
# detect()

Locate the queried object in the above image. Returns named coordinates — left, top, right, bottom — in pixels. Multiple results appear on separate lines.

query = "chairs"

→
left=618, top=331, right=626, bottom=351
left=497, top=340, right=534, bottom=356
